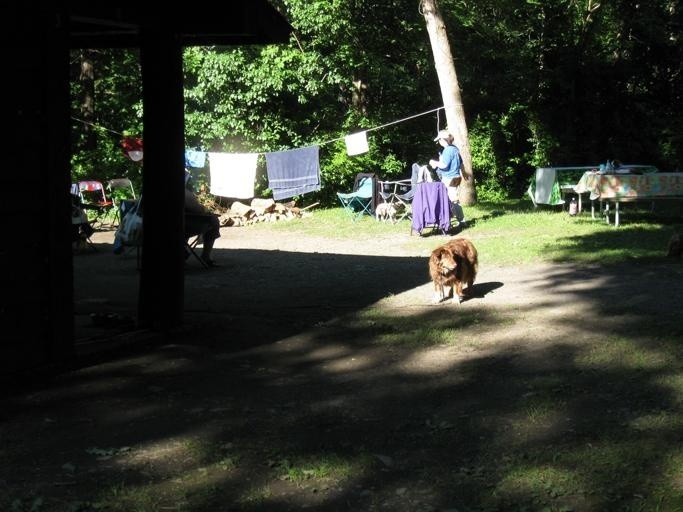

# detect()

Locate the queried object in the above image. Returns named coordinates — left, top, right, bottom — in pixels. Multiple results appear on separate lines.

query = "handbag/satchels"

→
left=116, top=213, right=143, bottom=246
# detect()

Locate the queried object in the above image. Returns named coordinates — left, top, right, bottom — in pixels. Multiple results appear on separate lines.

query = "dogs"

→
left=375, top=201, right=405, bottom=225
left=428, top=238, right=478, bottom=303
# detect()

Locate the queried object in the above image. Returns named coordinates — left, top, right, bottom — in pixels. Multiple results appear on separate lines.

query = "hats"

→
left=433, top=130, right=451, bottom=142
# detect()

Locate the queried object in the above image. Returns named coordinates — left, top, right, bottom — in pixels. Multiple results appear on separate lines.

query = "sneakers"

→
left=453, top=222, right=467, bottom=228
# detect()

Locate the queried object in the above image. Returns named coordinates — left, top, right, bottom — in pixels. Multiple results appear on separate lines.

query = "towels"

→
left=345, top=129, right=369, bottom=157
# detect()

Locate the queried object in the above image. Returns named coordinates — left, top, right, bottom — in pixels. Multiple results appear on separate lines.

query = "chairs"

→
left=335, top=170, right=378, bottom=221
left=64, top=175, right=220, bottom=275
left=411, top=182, right=451, bottom=237
left=378, top=163, right=429, bottom=224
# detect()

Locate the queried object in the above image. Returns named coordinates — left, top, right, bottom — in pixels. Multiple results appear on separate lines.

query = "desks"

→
left=573, top=168, right=682, bottom=230
left=524, top=165, right=659, bottom=210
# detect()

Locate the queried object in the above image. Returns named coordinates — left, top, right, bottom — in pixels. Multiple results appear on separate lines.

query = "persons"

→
left=429, top=130, right=472, bottom=229
left=185, top=168, right=224, bottom=271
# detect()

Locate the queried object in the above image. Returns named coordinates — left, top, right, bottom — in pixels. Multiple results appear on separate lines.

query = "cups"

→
left=600, top=163, right=605, bottom=171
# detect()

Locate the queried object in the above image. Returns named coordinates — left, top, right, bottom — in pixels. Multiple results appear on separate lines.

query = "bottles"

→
left=569, top=197, right=577, bottom=215
left=611, top=160, right=616, bottom=173
left=605, top=159, right=612, bottom=174
